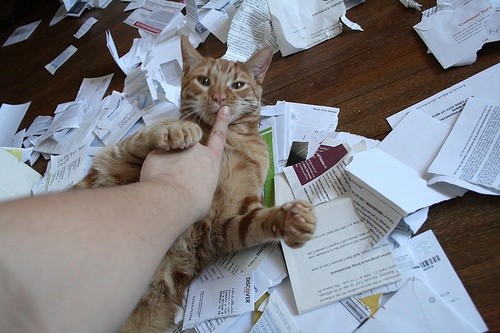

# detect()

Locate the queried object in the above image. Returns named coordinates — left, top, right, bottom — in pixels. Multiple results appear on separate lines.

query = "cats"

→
left=66, top=34, right=318, bottom=333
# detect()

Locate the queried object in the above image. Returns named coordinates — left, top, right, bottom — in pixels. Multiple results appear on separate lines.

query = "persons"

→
left=0, top=103, right=232, bottom=333
